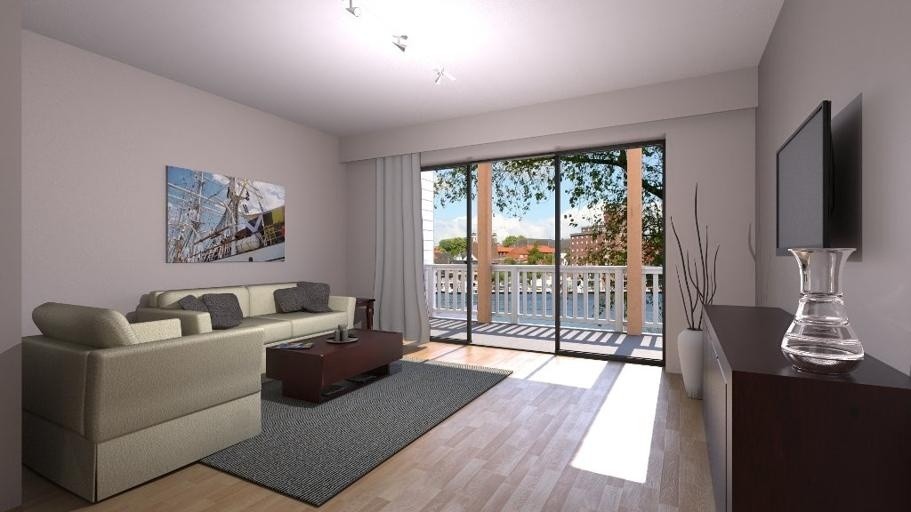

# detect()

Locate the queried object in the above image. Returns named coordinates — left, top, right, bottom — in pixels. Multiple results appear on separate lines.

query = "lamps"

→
left=344, top=1, right=458, bottom=84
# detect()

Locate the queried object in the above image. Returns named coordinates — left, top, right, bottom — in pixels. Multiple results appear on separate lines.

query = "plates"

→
left=324, top=336, right=361, bottom=345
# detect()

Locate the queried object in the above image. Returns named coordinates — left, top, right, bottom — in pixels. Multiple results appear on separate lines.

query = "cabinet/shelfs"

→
left=702, top=306, right=911, bottom=511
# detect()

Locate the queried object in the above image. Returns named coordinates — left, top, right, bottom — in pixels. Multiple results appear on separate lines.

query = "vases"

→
left=674, top=325, right=704, bottom=400
left=777, top=244, right=870, bottom=376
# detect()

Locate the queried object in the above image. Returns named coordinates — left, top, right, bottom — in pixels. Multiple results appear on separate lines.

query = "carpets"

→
left=196, top=349, right=512, bottom=507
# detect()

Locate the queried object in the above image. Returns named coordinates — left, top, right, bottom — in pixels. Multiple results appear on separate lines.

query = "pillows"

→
left=270, top=281, right=335, bottom=313
left=201, top=294, right=246, bottom=331
left=176, top=294, right=208, bottom=314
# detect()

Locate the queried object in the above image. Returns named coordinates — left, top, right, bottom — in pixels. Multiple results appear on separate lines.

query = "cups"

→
left=334, top=323, right=348, bottom=341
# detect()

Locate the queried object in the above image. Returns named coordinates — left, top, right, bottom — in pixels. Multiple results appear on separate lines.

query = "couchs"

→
left=139, top=282, right=358, bottom=372
left=22, top=300, right=263, bottom=506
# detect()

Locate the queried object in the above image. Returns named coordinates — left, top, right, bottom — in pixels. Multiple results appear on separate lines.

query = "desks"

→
left=353, top=296, right=375, bottom=332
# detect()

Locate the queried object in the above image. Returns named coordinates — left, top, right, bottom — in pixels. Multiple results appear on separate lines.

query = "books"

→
left=275, top=342, right=314, bottom=350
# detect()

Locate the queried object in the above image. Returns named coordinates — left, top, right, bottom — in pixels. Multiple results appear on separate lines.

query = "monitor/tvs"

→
left=775, top=100, right=832, bottom=256
